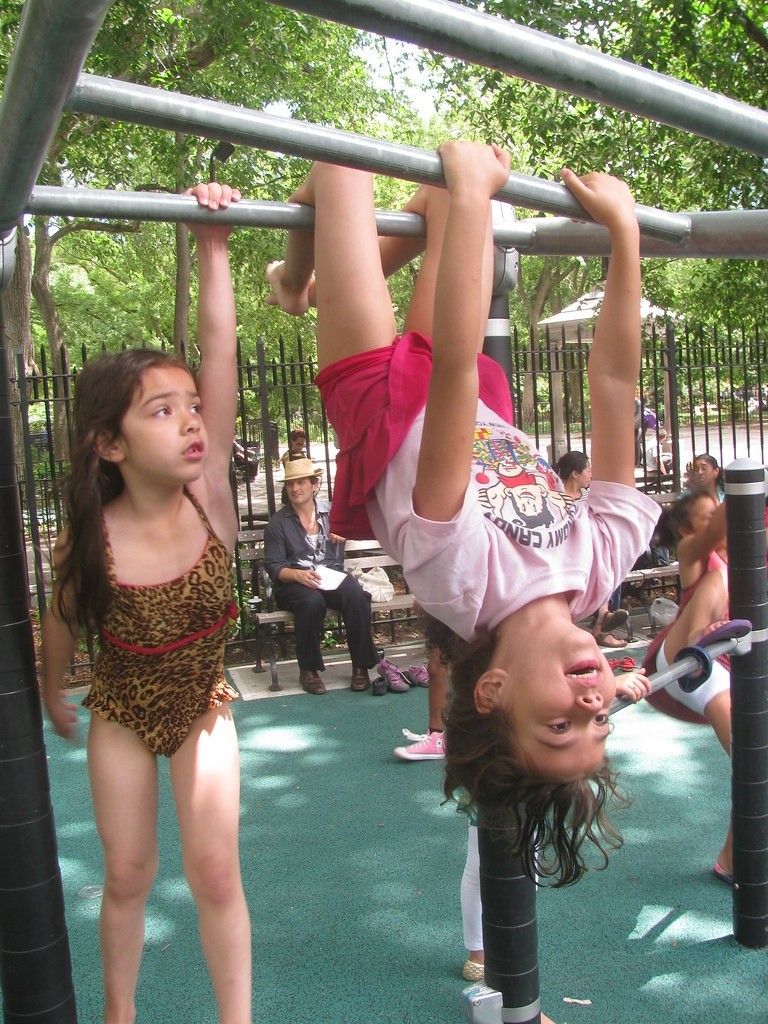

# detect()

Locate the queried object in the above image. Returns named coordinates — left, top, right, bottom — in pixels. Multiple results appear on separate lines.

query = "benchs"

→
left=237, top=493, right=679, bottom=691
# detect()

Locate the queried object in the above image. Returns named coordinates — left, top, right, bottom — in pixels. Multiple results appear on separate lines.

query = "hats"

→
left=276, top=459, right=323, bottom=483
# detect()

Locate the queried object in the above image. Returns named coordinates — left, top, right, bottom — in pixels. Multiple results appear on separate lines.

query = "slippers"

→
left=593, top=610, right=628, bottom=632
left=593, top=632, right=627, bottom=648
left=715, top=861, right=735, bottom=886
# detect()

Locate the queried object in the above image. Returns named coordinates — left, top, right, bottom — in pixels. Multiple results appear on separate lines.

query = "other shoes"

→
left=462, top=958, right=485, bottom=980
left=610, top=655, right=637, bottom=672
left=373, top=677, right=388, bottom=695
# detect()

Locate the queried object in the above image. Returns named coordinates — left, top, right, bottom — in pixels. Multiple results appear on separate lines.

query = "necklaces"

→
left=298, top=501, right=315, bottom=532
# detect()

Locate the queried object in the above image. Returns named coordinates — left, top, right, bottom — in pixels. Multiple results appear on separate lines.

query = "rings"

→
left=632, top=686, right=637, bottom=689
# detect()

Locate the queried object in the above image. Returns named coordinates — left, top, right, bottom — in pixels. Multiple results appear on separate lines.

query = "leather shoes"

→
left=352, top=666, right=369, bottom=690
left=299, top=670, right=326, bottom=695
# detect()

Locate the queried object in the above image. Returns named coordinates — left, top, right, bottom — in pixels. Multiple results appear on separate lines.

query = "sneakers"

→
left=376, top=658, right=410, bottom=692
left=408, top=664, right=430, bottom=687
left=394, top=729, right=446, bottom=760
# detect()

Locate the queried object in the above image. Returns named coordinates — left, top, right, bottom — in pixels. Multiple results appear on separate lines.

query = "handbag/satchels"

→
left=351, top=565, right=394, bottom=603
left=644, top=407, right=660, bottom=430
left=650, top=597, right=679, bottom=629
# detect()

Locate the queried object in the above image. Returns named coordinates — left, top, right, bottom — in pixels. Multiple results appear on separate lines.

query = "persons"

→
left=42, top=183, right=253, bottom=1024
left=265, top=141, right=663, bottom=887
left=232, top=393, right=768, bottom=981
